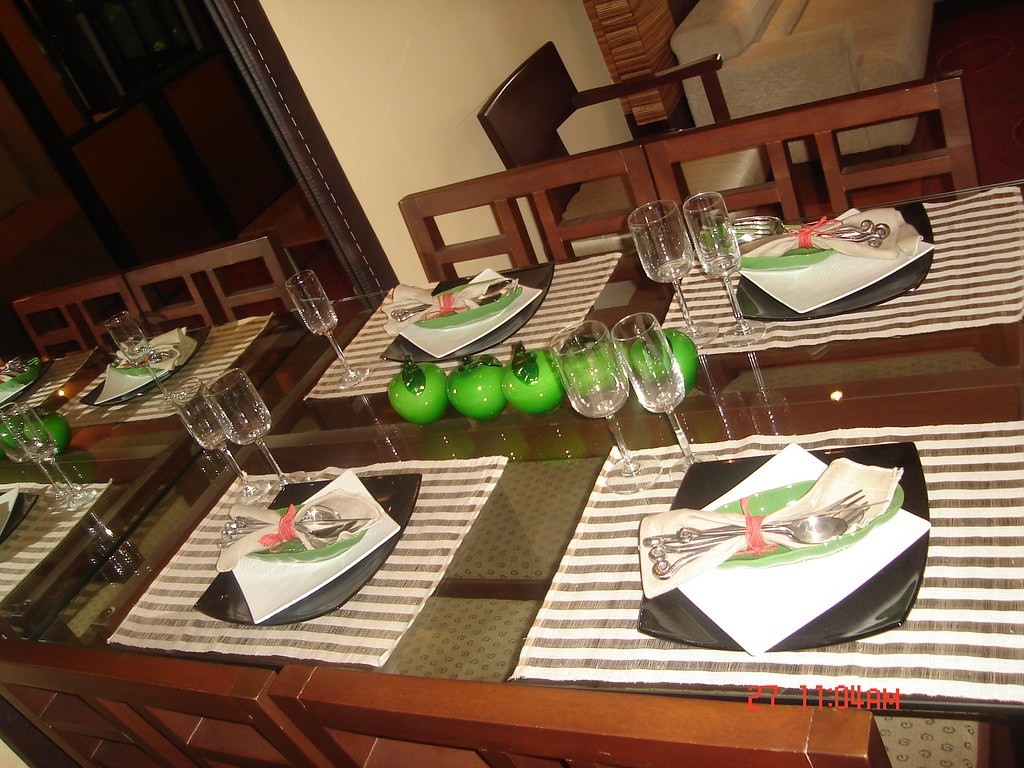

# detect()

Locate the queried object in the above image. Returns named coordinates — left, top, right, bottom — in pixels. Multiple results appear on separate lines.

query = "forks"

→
left=733, top=216, right=891, bottom=248
left=647, top=490, right=872, bottom=561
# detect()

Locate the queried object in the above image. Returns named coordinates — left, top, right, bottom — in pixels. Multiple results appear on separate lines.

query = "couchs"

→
left=669, top=1, right=934, bottom=155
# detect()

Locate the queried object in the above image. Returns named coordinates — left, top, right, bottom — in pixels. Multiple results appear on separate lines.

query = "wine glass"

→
left=697, top=356, right=741, bottom=441
left=104, top=310, right=176, bottom=411
left=627, top=199, right=720, bottom=349
left=2, top=401, right=97, bottom=510
left=610, top=311, right=717, bottom=489
left=745, top=351, right=795, bottom=436
left=549, top=320, right=664, bottom=496
left=683, top=191, right=768, bottom=349
left=202, top=368, right=310, bottom=499
left=0, top=412, right=82, bottom=509
left=169, top=375, right=271, bottom=506
left=284, top=269, right=369, bottom=391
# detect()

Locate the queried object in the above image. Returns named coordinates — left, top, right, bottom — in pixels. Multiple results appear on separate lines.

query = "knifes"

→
left=223, top=520, right=357, bottom=537
left=391, top=278, right=512, bottom=318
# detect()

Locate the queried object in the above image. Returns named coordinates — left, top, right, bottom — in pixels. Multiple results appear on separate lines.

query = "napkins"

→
left=215, top=489, right=382, bottom=572
left=381, top=278, right=519, bottom=337
left=116, top=327, right=187, bottom=371
left=637, top=458, right=903, bottom=599
left=738, top=207, right=923, bottom=261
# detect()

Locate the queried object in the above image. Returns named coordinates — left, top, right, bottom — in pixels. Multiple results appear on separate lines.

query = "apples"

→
left=387, top=325, right=698, bottom=424
left=0, top=412, right=70, bottom=452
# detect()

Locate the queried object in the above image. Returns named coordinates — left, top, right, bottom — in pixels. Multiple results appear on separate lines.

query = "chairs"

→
left=1, top=40, right=1019, bottom=768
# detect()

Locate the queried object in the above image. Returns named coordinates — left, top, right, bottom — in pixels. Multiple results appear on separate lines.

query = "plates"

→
left=232, top=469, right=402, bottom=625
left=0, top=360, right=55, bottom=406
left=193, top=473, right=422, bottom=627
left=93, top=326, right=187, bottom=404
left=0, top=491, right=40, bottom=545
left=79, top=324, right=211, bottom=407
left=736, top=200, right=935, bottom=322
left=737, top=207, right=936, bottom=314
left=636, top=441, right=930, bottom=652
left=398, top=267, right=543, bottom=359
left=378, top=259, right=554, bottom=364
left=0, top=486, right=19, bottom=536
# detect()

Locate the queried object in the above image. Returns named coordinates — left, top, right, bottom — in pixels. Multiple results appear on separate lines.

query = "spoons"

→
left=229, top=505, right=341, bottom=526
left=676, top=516, right=848, bottom=544
left=643, top=504, right=865, bottom=524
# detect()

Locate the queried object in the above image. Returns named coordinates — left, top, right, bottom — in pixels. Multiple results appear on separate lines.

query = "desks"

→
left=238, top=181, right=330, bottom=300
left=0, top=177, right=1023, bottom=768
